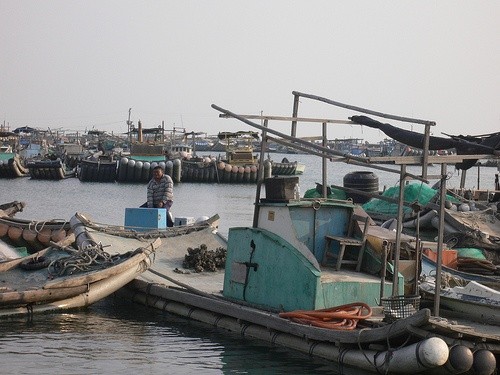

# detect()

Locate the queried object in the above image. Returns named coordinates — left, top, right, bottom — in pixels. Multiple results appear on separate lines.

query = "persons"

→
left=139, top=166, right=175, bottom=227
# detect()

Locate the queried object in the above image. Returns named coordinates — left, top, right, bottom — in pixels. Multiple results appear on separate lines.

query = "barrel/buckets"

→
left=344, top=171, right=379, bottom=203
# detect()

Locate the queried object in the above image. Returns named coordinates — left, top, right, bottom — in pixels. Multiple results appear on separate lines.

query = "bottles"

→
left=293, top=183, right=300, bottom=202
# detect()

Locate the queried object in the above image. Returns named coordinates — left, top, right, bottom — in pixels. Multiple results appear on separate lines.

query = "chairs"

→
left=321, top=213, right=371, bottom=271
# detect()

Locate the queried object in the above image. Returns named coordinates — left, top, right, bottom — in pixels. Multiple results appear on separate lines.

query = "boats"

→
left=70, top=90, right=500, bottom=375
left=1, top=108, right=305, bottom=183
left=305, top=136, right=499, bottom=167
left=0, top=233, right=163, bottom=319
left=1, top=199, right=221, bottom=256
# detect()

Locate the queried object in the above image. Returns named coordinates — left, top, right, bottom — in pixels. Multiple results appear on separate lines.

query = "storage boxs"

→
left=174, top=217, right=193, bottom=227
left=123, top=207, right=167, bottom=231
